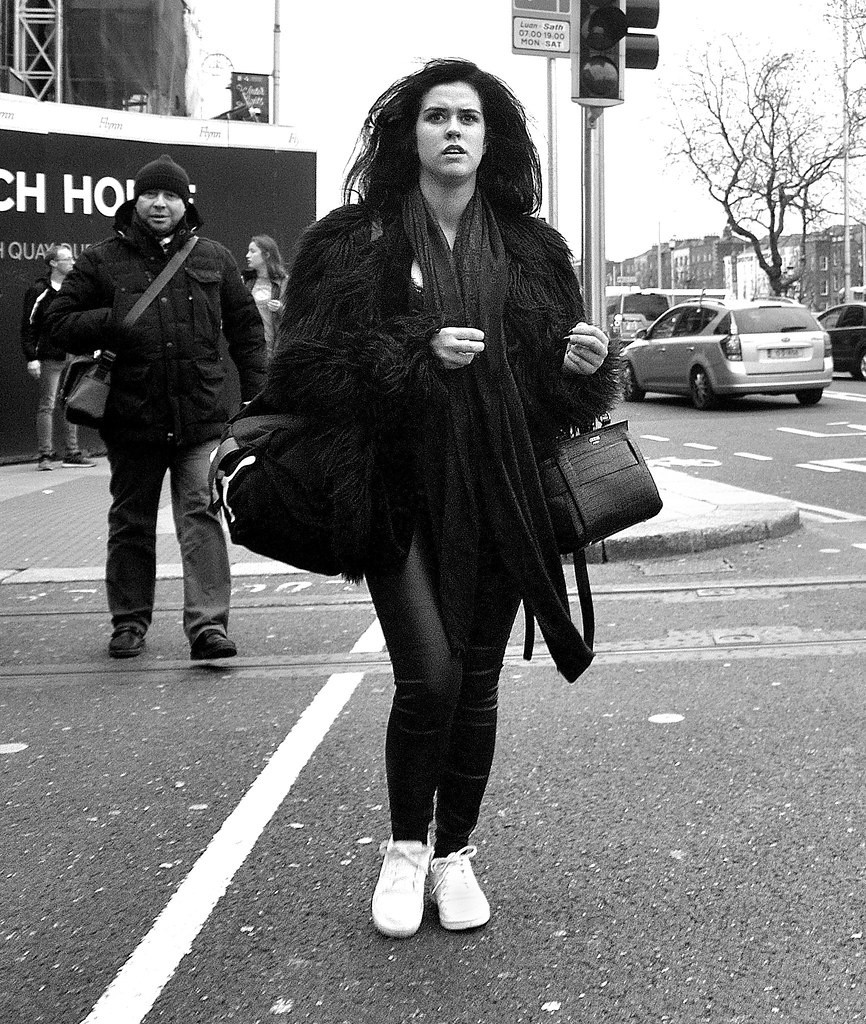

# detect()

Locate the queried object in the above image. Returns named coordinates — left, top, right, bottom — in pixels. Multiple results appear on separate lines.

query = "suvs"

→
left=617, top=296, right=834, bottom=410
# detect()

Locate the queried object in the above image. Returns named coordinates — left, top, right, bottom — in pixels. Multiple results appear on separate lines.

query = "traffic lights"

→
left=624, top=0, right=660, bottom=70
left=571, top=1, right=627, bottom=106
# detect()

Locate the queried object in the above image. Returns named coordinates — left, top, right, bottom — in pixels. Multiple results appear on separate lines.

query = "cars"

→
left=815, top=302, right=866, bottom=382
left=603, top=287, right=732, bottom=348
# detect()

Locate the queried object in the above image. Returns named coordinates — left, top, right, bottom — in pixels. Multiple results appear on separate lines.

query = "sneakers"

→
left=191, top=630, right=237, bottom=661
left=371, top=834, right=435, bottom=939
left=109, top=627, right=144, bottom=657
left=430, top=844, right=491, bottom=931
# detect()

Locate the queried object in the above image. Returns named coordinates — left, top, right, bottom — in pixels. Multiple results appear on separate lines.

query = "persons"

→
left=20, top=240, right=97, bottom=472
left=266, top=57, right=625, bottom=940
left=41, top=154, right=269, bottom=661
left=241, top=231, right=290, bottom=347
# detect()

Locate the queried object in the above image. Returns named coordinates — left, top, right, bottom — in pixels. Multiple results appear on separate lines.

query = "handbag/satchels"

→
left=530, top=405, right=663, bottom=556
left=207, top=369, right=365, bottom=577
left=57, top=344, right=119, bottom=427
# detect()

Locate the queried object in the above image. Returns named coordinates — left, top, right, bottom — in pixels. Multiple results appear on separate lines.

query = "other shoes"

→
left=38, top=456, right=53, bottom=471
left=62, top=454, right=96, bottom=468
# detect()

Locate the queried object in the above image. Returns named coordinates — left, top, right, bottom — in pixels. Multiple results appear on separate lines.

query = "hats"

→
left=133, top=154, right=191, bottom=200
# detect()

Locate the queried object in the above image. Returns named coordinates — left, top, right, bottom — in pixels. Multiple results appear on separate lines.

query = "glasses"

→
left=54, top=257, right=75, bottom=263
